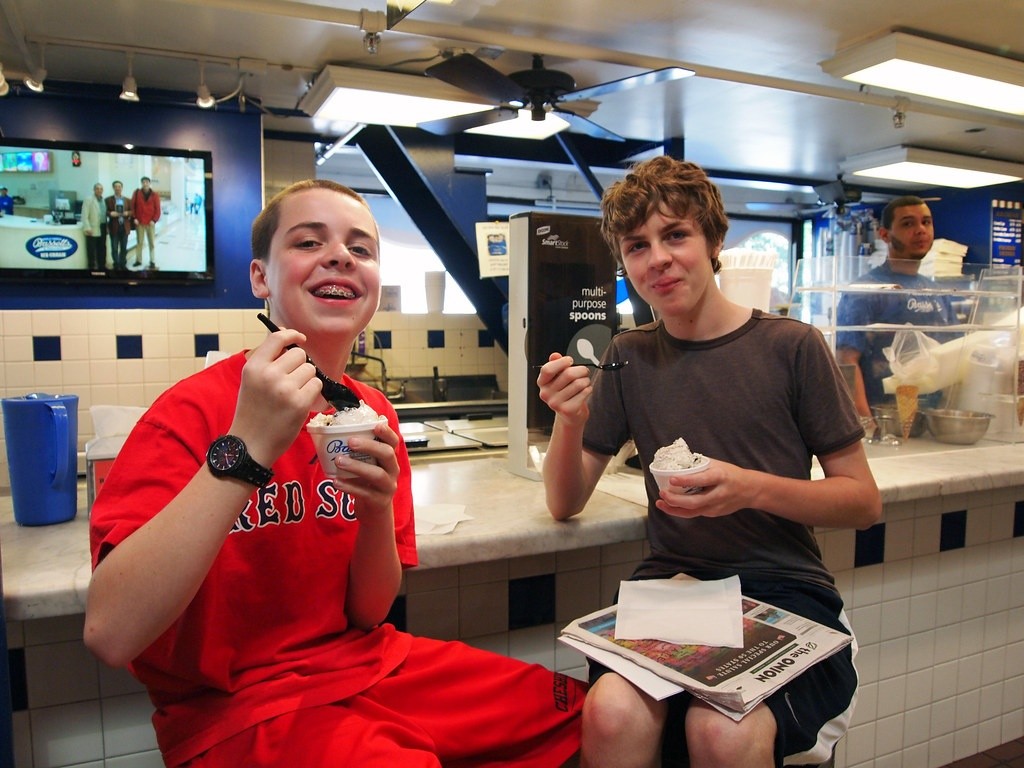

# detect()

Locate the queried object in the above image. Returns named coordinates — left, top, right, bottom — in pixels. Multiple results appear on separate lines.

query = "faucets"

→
left=350, top=350, right=408, bottom=401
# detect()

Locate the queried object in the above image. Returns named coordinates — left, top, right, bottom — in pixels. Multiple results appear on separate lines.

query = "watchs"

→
left=206, top=435, right=271, bottom=488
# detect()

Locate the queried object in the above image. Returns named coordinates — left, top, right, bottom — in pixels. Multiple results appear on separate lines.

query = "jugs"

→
left=1, top=392, right=79, bottom=526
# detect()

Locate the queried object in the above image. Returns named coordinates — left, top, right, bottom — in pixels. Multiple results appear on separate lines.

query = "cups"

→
left=815, top=206, right=871, bottom=305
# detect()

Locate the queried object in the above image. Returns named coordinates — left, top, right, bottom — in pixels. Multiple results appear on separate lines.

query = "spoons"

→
left=531, top=360, right=632, bottom=370
left=257, top=312, right=361, bottom=410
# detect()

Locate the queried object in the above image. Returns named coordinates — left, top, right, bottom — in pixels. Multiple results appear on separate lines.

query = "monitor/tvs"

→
left=49, top=189, right=77, bottom=218
left=0, top=136, right=215, bottom=286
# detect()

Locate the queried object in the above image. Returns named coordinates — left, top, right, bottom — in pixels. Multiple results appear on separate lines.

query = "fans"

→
left=414, top=46, right=697, bottom=142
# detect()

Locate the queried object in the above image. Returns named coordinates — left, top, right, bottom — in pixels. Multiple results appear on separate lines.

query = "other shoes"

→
left=133, top=261, right=141, bottom=266
left=149, top=262, right=155, bottom=269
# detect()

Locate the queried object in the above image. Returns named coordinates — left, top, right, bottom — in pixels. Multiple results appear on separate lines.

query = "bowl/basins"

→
left=870, top=404, right=996, bottom=445
left=648, top=456, right=710, bottom=498
left=306, top=420, right=388, bottom=480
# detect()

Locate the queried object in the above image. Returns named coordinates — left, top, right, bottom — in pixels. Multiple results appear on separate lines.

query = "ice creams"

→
left=650, top=436, right=703, bottom=471
left=308, top=399, right=385, bottom=426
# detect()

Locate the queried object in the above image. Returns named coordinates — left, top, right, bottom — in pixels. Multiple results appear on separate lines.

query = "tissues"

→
left=83, top=404, right=150, bottom=520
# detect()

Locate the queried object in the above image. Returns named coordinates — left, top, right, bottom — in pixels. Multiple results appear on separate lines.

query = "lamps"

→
left=20, top=41, right=50, bottom=96
left=118, top=52, right=141, bottom=103
left=837, top=144, right=1024, bottom=190
left=815, top=26, right=1024, bottom=121
left=190, top=61, right=217, bottom=109
left=295, top=61, right=600, bottom=145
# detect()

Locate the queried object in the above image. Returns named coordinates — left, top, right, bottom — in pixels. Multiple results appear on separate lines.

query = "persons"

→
left=85, top=178, right=592, bottom=768
left=131, top=177, right=160, bottom=268
left=837, top=195, right=964, bottom=431
left=105, top=180, right=132, bottom=271
left=537, top=156, right=882, bottom=768
left=0, top=188, right=14, bottom=215
left=82, top=183, right=108, bottom=275
